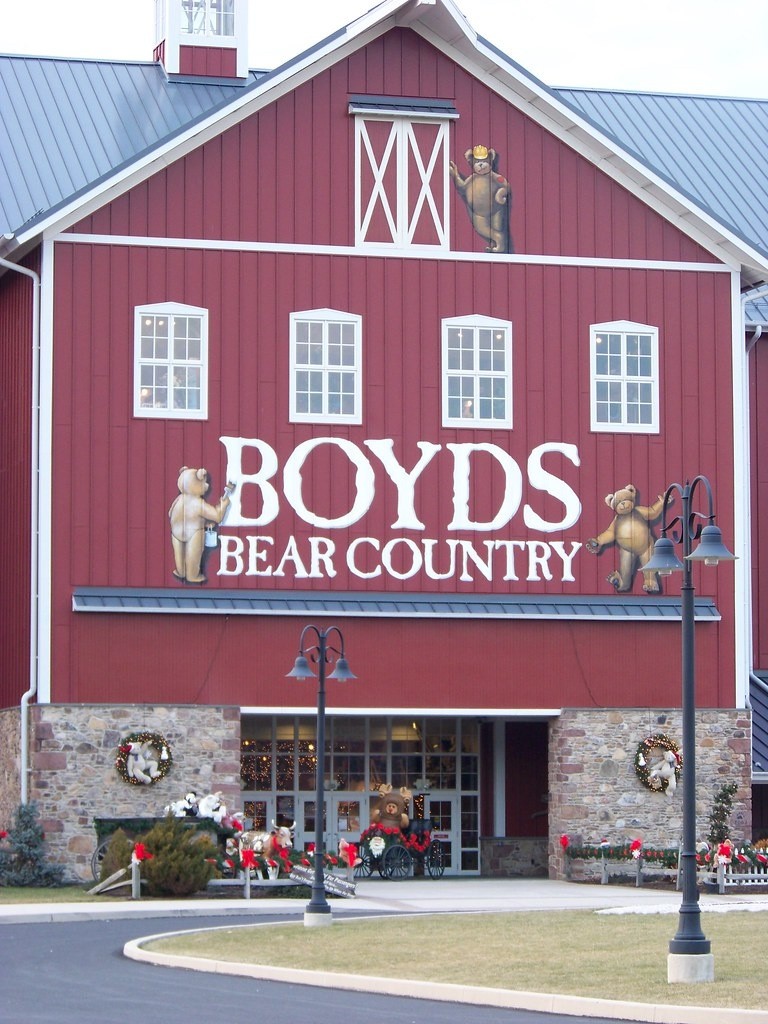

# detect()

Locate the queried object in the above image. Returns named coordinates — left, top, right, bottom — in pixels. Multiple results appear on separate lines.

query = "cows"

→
left=240, top=820, right=297, bottom=880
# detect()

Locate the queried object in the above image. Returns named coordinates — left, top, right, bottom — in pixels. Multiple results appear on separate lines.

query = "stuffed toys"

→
left=164, top=790, right=246, bottom=830
left=695, top=842, right=716, bottom=885
left=373, top=784, right=412, bottom=828
left=127, top=740, right=162, bottom=784
left=647, top=751, right=680, bottom=797
left=339, top=839, right=362, bottom=867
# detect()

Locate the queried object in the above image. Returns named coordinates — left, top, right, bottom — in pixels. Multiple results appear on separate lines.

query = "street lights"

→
left=637, top=473, right=739, bottom=986
left=284, top=621, right=357, bottom=931
